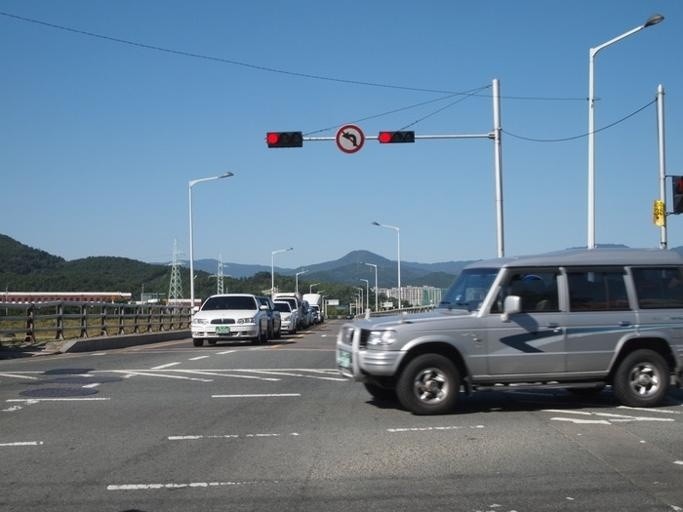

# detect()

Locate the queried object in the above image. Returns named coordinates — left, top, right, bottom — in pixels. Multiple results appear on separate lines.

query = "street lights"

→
left=295, top=268, right=311, bottom=292
left=359, top=278, right=369, bottom=309
left=370, top=220, right=402, bottom=311
left=584, top=12, right=664, bottom=248
left=186, top=170, right=235, bottom=314
left=308, top=281, right=328, bottom=317
left=358, top=260, right=379, bottom=312
left=270, top=247, right=294, bottom=292
left=349, top=286, right=364, bottom=316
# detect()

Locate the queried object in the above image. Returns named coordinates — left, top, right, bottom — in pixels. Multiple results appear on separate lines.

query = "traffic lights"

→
left=377, top=130, right=415, bottom=144
left=671, top=175, right=683, bottom=213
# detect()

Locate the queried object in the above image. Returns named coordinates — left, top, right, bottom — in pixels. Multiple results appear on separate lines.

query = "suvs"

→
left=330, top=244, right=682, bottom=422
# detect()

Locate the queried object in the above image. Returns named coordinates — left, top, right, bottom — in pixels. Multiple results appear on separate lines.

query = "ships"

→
left=265, top=130, right=303, bottom=149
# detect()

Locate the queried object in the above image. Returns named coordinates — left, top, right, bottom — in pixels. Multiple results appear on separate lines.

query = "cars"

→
left=345, top=314, right=354, bottom=320
left=185, top=292, right=271, bottom=346
left=271, top=290, right=326, bottom=335
left=254, top=295, right=284, bottom=339
